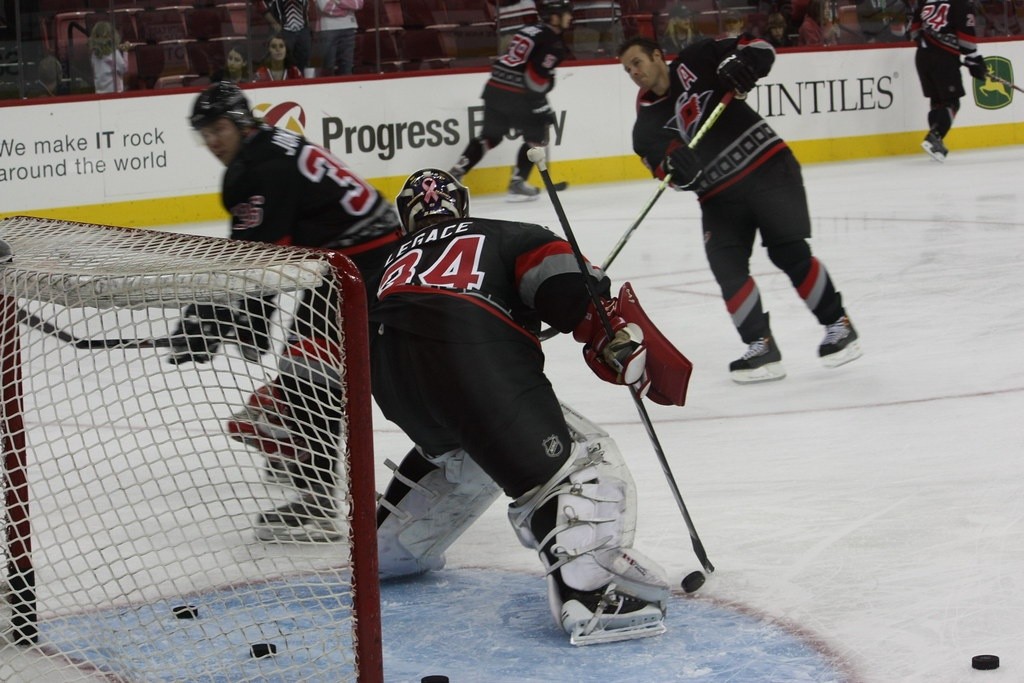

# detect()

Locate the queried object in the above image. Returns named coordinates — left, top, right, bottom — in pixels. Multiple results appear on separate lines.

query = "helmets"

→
left=397, top=169, right=469, bottom=233
left=539, top=0, right=574, bottom=21
left=190, top=81, right=253, bottom=130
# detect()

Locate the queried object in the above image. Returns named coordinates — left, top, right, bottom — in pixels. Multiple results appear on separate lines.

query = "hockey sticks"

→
left=16, top=308, right=238, bottom=350
left=552, top=180, right=567, bottom=191
left=526, top=144, right=715, bottom=574
left=600, top=89, right=737, bottom=271
left=964, top=60, right=1024, bottom=94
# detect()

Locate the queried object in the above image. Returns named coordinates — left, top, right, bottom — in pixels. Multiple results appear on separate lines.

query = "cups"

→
left=304, top=67, right=315, bottom=78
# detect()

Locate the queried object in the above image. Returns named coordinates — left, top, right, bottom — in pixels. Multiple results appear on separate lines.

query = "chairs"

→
left=34, top=0, right=1024, bottom=100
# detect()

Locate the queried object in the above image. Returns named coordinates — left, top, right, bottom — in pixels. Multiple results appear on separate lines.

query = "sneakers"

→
left=922, top=129, right=949, bottom=162
left=561, top=583, right=665, bottom=646
left=255, top=485, right=343, bottom=543
left=504, top=178, right=540, bottom=204
left=819, top=314, right=865, bottom=368
left=730, top=335, right=787, bottom=382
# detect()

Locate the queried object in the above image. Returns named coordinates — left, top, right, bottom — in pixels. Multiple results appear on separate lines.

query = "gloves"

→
left=964, top=52, right=990, bottom=81
left=663, top=146, right=703, bottom=190
left=715, top=50, right=758, bottom=93
left=601, top=331, right=635, bottom=374
left=168, top=297, right=274, bottom=369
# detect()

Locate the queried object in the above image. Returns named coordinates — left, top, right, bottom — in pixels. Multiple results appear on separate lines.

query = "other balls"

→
left=249, top=642, right=278, bottom=659
left=421, top=675, right=449, bottom=683
left=173, top=603, right=200, bottom=619
left=681, top=571, right=706, bottom=593
left=972, top=654, right=1000, bottom=670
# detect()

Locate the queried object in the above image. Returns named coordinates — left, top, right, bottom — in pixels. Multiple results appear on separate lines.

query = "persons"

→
left=856, top=0, right=915, bottom=44
left=376, top=169, right=666, bottom=636
left=208, top=45, right=252, bottom=84
left=757, top=12, right=798, bottom=47
left=660, top=4, right=706, bottom=53
left=573, top=0, right=625, bottom=60
left=252, top=34, right=302, bottom=81
left=799, top=0, right=839, bottom=46
left=250, top=0, right=313, bottom=78
left=312, top=0, right=364, bottom=78
left=447, top=0, right=575, bottom=196
left=712, top=10, right=744, bottom=41
left=27, top=57, right=62, bottom=98
left=916, top=0, right=987, bottom=157
left=486, top=0, right=541, bottom=56
left=88, top=21, right=130, bottom=94
left=168, top=82, right=408, bottom=528
left=616, top=35, right=858, bottom=371
